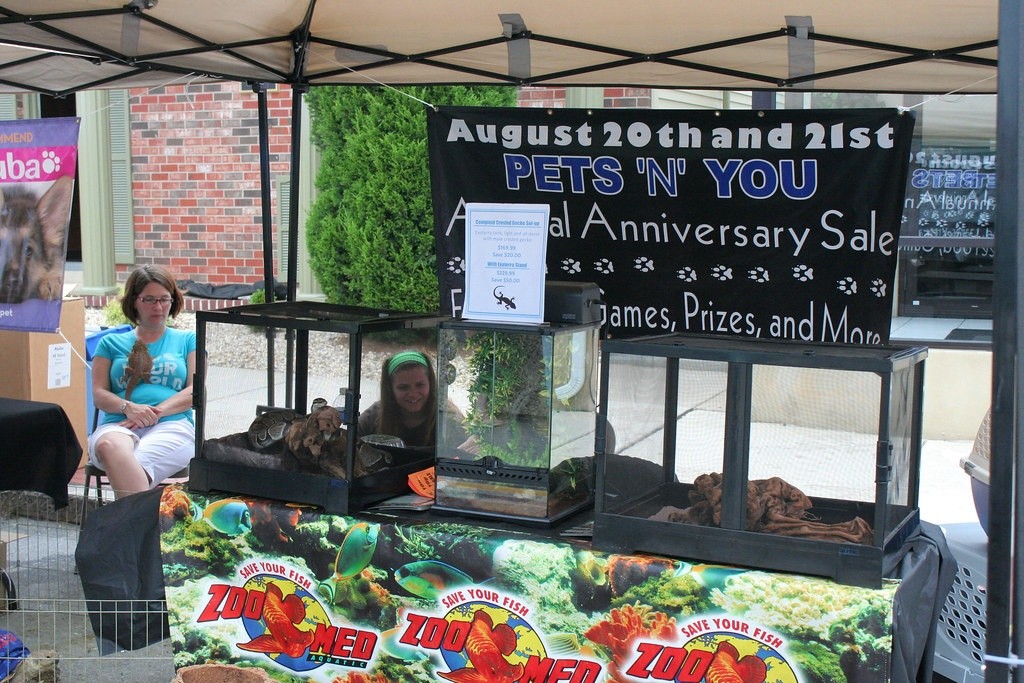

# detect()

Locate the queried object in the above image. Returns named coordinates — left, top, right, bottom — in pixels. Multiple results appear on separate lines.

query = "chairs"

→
left=83, top=325, right=190, bottom=502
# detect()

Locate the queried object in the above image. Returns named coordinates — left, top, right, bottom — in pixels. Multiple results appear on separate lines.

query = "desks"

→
left=158, top=481, right=958, bottom=683
left=0, top=397, right=84, bottom=610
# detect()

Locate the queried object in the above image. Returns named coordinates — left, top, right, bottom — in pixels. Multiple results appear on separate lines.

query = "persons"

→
left=88, top=265, right=208, bottom=501
left=355, top=351, right=481, bottom=455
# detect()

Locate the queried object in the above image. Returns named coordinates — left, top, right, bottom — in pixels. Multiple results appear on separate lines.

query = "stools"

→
left=73, top=466, right=110, bottom=576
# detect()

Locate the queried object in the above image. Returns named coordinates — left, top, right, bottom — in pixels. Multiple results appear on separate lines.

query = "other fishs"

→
left=320, top=522, right=381, bottom=600
left=191, top=498, right=253, bottom=538
left=281, top=556, right=334, bottom=612
left=394, top=561, right=496, bottom=603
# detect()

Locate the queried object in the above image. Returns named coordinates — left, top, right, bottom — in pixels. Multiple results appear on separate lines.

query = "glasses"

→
left=137, top=296, right=174, bottom=305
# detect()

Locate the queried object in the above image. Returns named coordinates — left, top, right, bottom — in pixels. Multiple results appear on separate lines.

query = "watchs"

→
left=120, top=401, right=130, bottom=413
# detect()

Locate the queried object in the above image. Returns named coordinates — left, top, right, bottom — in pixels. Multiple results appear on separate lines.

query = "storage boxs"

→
left=188, top=300, right=452, bottom=514
left=435, top=319, right=600, bottom=531
left=0, top=296, right=89, bottom=469
left=933, top=521, right=1012, bottom=683
left=590, top=331, right=929, bottom=589
